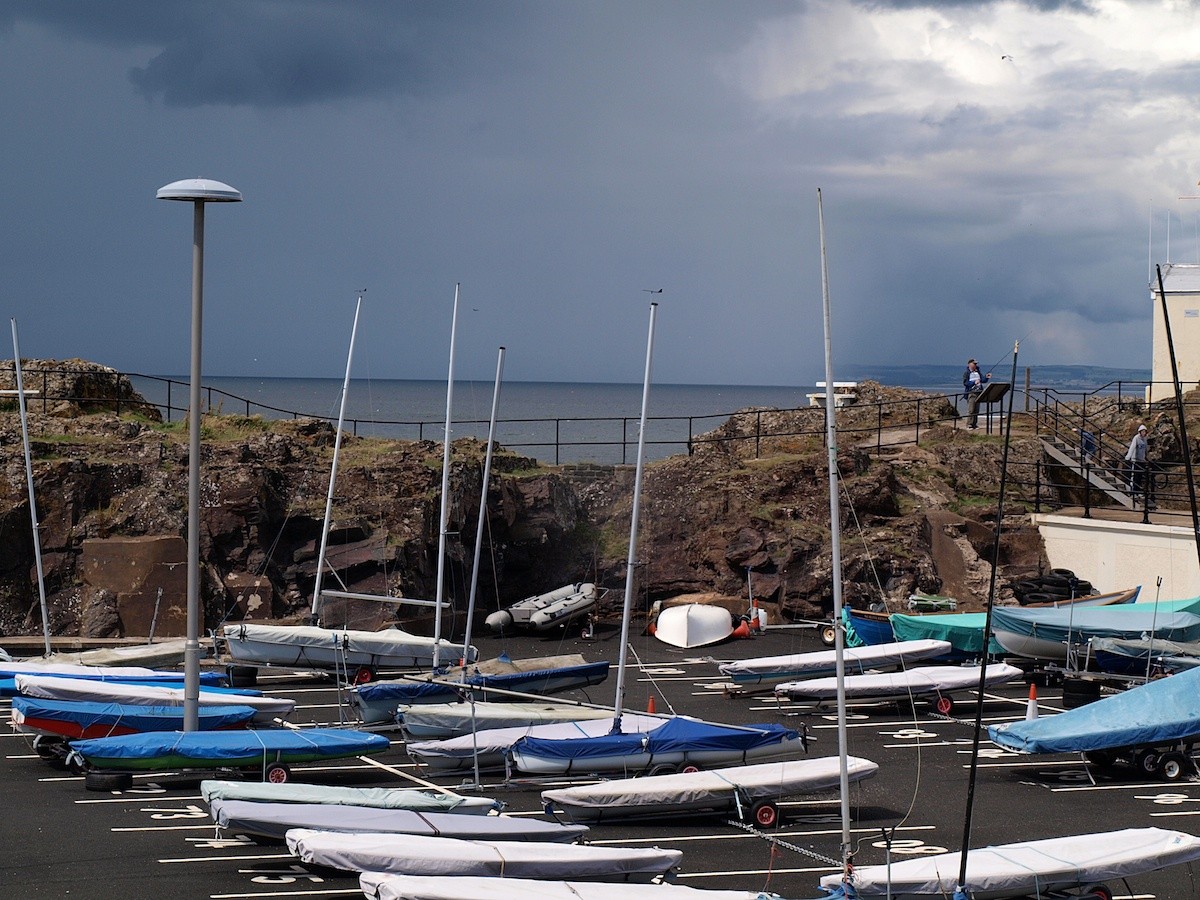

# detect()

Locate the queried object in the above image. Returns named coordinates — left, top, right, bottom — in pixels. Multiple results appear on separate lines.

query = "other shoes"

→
left=1130, top=494, right=1144, bottom=499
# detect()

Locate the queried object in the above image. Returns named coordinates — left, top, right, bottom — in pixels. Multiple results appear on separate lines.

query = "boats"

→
left=0, top=661, right=389, bottom=787
left=989, top=665, right=1200, bottom=775
left=717, top=636, right=1024, bottom=714
left=538, top=756, right=880, bottom=827
left=653, top=604, right=733, bottom=649
left=203, top=780, right=780, bottom=900
left=406, top=713, right=695, bottom=774
left=846, top=587, right=1200, bottom=675
left=484, top=582, right=598, bottom=633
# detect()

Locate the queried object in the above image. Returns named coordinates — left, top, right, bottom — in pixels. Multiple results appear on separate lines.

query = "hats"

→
left=968, top=358, right=978, bottom=363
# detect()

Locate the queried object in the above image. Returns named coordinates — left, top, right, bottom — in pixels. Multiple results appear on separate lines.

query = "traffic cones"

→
left=747, top=599, right=766, bottom=636
left=641, top=618, right=656, bottom=636
left=647, top=693, right=655, bottom=713
left=733, top=620, right=750, bottom=638
left=1026, top=683, right=1038, bottom=720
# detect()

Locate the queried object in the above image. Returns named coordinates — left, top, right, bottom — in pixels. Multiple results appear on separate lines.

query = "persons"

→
left=1072, top=426, right=1096, bottom=460
left=963, top=359, right=992, bottom=430
left=1125, top=424, right=1148, bottom=499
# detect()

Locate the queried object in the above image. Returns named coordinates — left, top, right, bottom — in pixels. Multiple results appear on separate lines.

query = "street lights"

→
left=156, top=178, right=244, bottom=731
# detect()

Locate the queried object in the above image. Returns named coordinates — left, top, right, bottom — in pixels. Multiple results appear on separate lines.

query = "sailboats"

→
left=339, top=276, right=610, bottom=724
left=223, top=299, right=480, bottom=667
left=394, top=346, right=617, bottom=739
left=0, top=317, right=217, bottom=666
left=818, top=184, right=1200, bottom=900
left=509, top=300, right=807, bottom=777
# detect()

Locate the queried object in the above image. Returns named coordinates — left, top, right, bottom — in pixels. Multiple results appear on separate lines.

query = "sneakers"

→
left=966, top=426, right=980, bottom=431
